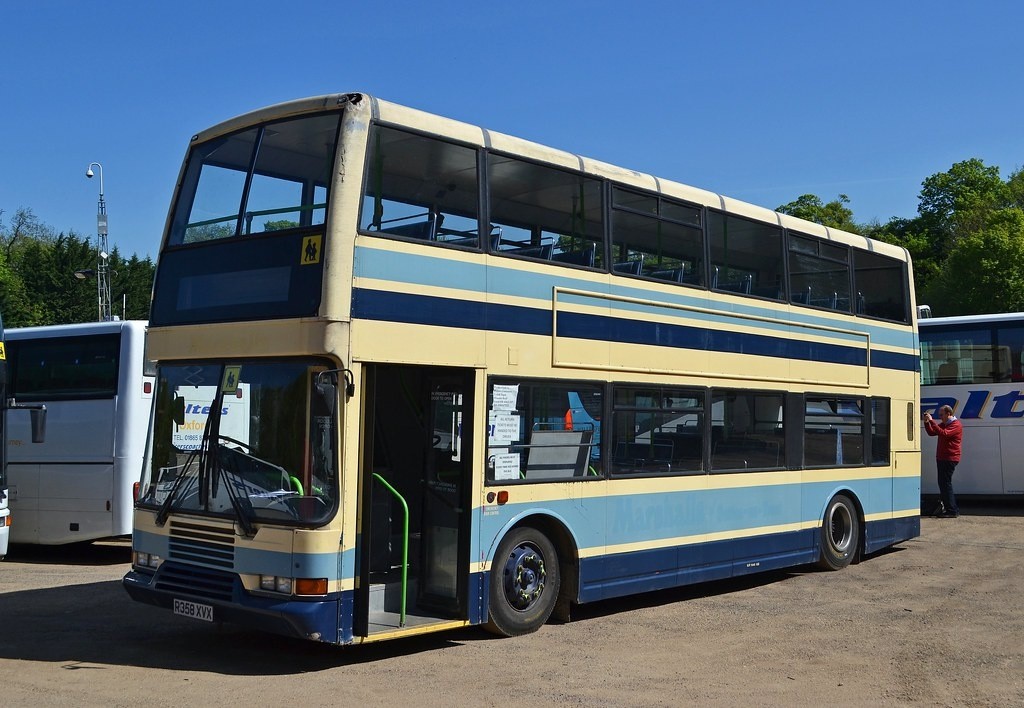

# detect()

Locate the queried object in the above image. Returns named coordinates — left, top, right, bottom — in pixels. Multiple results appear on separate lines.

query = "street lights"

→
left=73, top=162, right=118, bottom=323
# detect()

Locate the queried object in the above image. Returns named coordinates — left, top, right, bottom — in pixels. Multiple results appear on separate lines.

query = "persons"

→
left=923, top=405, right=963, bottom=518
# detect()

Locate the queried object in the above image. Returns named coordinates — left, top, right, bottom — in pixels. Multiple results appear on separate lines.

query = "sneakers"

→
left=936, top=508, right=959, bottom=518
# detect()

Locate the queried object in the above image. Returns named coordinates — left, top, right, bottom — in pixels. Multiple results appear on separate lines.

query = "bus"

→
left=510, top=305, right=1024, bottom=501
left=121, top=94, right=923, bottom=648
left=0, top=319, right=250, bottom=561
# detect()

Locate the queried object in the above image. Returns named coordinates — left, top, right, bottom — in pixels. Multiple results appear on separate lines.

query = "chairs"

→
left=936, top=364, right=957, bottom=383
left=587, top=413, right=874, bottom=474
left=363, top=207, right=867, bottom=328
